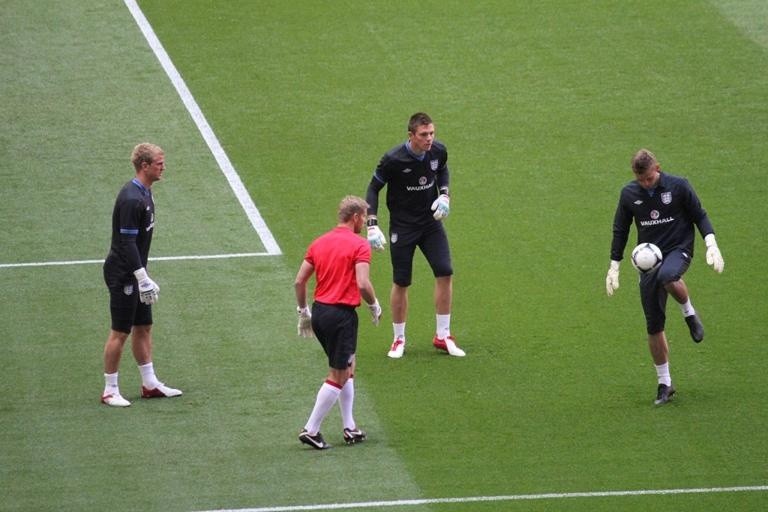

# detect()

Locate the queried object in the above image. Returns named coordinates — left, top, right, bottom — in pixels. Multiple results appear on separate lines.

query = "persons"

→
left=101, top=145, right=182, bottom=408
left=295, top=195, right=383, bottom=451
left=603, top=149, right=724, bottom=406
left=367, top=114, right=467, bottom=361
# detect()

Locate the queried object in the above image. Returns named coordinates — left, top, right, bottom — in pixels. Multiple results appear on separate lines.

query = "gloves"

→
left=366, top=217, right=387, bottom=252
left=296, top=305, right=313, bottom=338
left=431, top=188, right=450, bottom=220
left=606, top=261, right=620, bottom=296
left=367, top=299, right=382, bottom=327
left=705, top=234, right=724, bottom=273
left=133, top=268, right=160, bottom=305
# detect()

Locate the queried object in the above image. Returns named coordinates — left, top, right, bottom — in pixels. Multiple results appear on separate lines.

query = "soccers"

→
left=630, top=243, right=662, bottom=275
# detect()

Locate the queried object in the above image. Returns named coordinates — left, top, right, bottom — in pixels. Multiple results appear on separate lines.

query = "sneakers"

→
left=343, top=428, right=367, bottom=445
left=297, top=427, right=332, bottom=450
left=684, top=312, right=704, bottom=343
left=387, top=338, right=405, bottom=359
left=141, top=384, right=183, bottom=398
left=653, top=380, right=676, bottom=406
left=102, top=393, right=131, bottom=407
left=432, top=334, right=465, bottom=357
